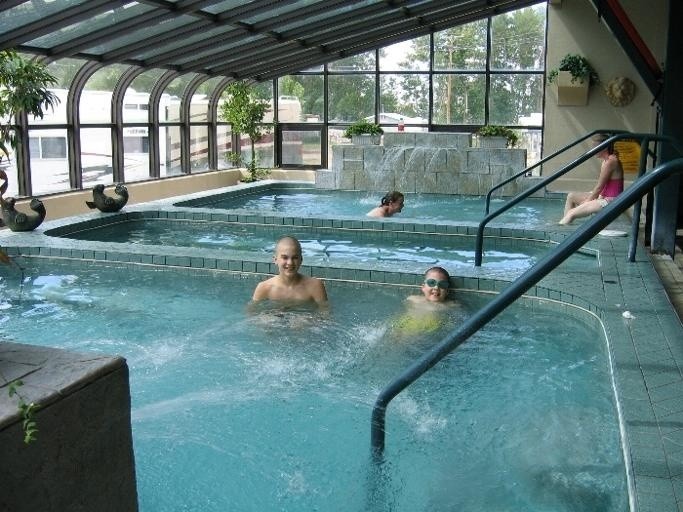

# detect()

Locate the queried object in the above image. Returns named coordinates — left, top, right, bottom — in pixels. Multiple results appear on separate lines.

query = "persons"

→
left=391, top=266, right=461, bottom=341
left=365, top=190, right=405, bottom=217
left=557, top=135, right=623, bottom=225
left=243, top=235, right=331, bottom=317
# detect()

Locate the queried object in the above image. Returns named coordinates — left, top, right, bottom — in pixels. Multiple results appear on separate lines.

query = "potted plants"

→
left=216, top=78, right=279, bottom=185
left=341, top=116, right=385, bottom=146
left=471, top=125, right=518, bottom=149
left=547, top=53, right=602, bottom=106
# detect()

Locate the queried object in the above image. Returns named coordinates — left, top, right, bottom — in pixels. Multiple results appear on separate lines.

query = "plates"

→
left=601, top=230, right=628, bottom=238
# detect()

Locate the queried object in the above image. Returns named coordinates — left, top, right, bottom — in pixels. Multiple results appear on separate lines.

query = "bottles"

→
left=398, top=118, right=405, bottom=131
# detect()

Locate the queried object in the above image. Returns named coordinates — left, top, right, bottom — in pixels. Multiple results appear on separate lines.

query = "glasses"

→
left=427, top=278, right=449, bottom=289
